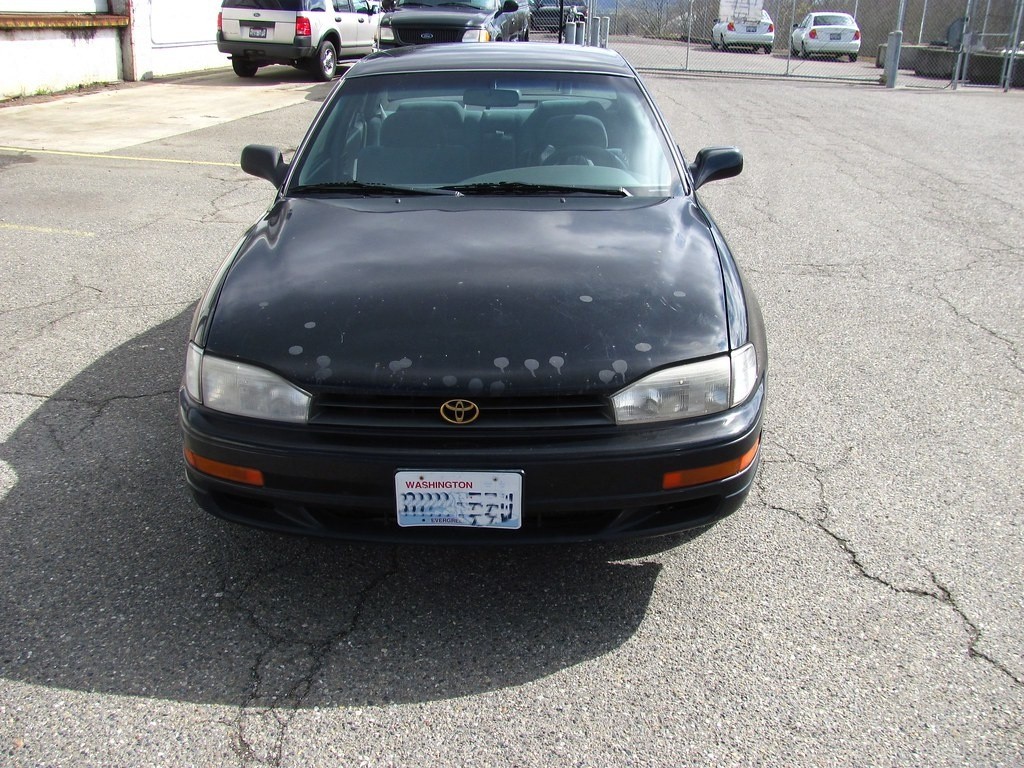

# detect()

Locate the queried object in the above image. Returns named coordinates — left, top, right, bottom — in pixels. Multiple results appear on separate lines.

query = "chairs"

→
left=357, top=111, right=475, bottom=186
left=521, top=115, right=631, bottom=170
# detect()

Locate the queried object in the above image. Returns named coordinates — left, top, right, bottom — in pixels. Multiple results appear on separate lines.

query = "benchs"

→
left=374, top=99, right=634, bottom=173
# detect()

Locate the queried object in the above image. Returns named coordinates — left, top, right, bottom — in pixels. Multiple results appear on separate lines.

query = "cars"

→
left=175, top=42, right=769, bottom=548
left=710, top=9, right=774, bottom=54
left=790, top=12, right=862, bottom=62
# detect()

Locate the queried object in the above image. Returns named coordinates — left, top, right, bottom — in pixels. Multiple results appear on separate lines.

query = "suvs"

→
left=369, top=0, right=532, bottom=54
left=216, top=0, right=397, bottom=83
left=527, top=0, right=589, bottom=36
